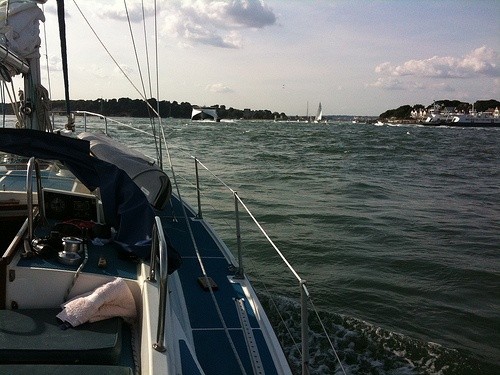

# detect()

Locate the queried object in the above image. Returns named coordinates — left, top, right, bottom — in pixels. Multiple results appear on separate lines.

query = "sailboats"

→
left=314, top=102, right=323, bottom=124
left=1, top=1, right=346, bottom=374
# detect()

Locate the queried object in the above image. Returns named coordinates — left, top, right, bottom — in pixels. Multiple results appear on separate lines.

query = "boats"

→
left=420, top=112, right=500, bottom=127
